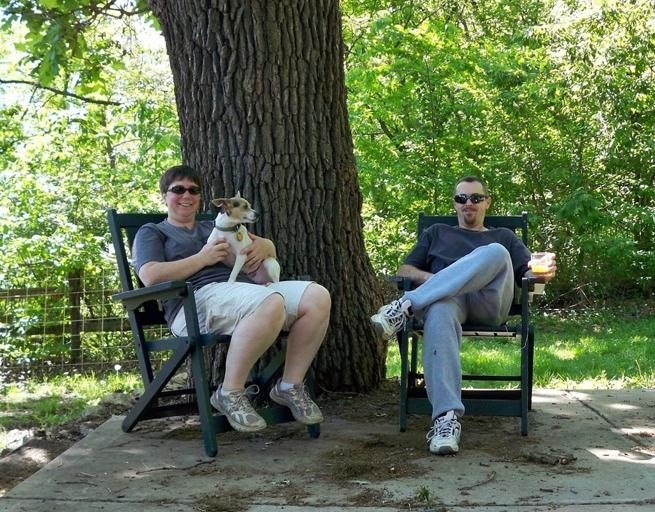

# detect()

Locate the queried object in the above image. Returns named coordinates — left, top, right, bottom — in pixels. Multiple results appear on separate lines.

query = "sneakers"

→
left=426, top=411, right=461, bottom=454
left=370, top=300, right=414, bottom=341
left=210, top=384, right=267, bottom=433
left=270, top=378, right=324, bottom=425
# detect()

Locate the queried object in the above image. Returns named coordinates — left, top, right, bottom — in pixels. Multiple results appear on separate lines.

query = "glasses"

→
left=454, top=193, right=485, bottom=204
left=167, top=186, right=201, bottom=195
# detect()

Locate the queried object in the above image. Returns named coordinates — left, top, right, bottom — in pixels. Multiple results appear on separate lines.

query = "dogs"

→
left=206, top=190, right=281, bottom=289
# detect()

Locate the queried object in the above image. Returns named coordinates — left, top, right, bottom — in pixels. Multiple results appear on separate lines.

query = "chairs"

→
left=108, top=209, right=320, bottom=457
left=390, top=212, right=546, bottom=436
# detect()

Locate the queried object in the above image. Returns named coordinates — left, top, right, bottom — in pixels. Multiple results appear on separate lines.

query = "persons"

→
left=369, top=176, right=558, bottom=454
left=131, top=164, right=332, bottom=433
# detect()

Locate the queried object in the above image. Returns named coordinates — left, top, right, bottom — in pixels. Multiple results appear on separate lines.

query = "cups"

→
left=531, top=253, right=551, bottom=276
